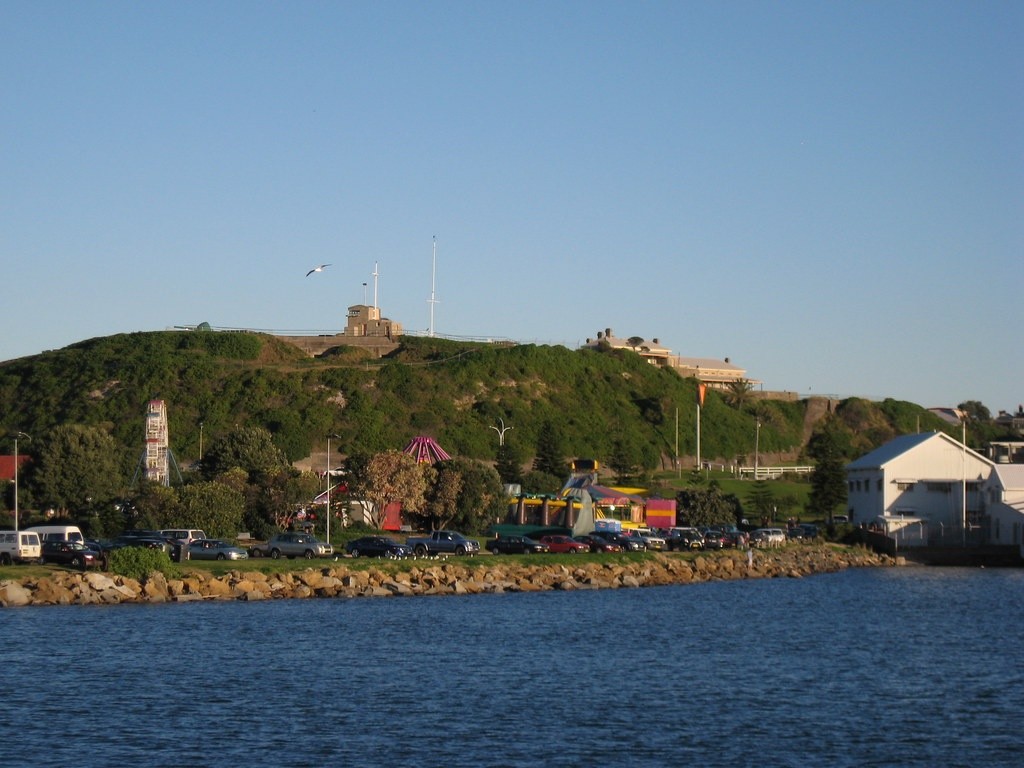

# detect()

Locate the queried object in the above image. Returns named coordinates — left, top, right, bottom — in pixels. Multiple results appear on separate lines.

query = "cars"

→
left=39, top=541, right=96, bottom=568
left=186, top=538, right=249, bottom=562
left=248, top=541, right=270, bottom=559
left=84, top=530, right=186, bottom=559
left=345, top=537, right=414, bottom=559
left=589, top=530, right=646, bottom=553
left=832, top=514, right=849, bottom=524
left=572, top=534, right=623, bottom=555
left=540, top=532, right=590, bottom=554
left=485, top=534, right=550, bottom=555
left=268, top=531, right=334, bottom=559
left=658, top=524, right=818, bottom=550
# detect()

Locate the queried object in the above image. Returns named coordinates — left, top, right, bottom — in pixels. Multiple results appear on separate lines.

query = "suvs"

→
left=628, top=528, right=666, bottom=551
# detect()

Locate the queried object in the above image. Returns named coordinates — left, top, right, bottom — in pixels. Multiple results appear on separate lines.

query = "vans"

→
left=24, top=526, right=83, bottom=550
left=154, top=529, right=208, bottom=544
left=0, top=531, right=41, bottom=567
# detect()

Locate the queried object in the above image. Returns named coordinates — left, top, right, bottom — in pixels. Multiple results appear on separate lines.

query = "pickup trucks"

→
left=405, top=530, right=481, bottom=558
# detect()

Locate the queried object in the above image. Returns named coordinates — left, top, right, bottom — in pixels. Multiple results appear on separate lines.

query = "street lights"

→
left=959, top=414, right=971, bottom=551
left=325, top=433, right=342, bottom=544
left=7, top=431, right=31, bottom=531
left=487, top=425, right=514, bottom=446
